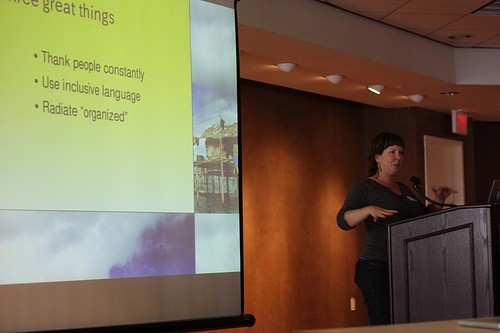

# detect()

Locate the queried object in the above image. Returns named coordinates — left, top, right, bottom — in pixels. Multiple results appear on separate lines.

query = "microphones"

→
left=410, top=176, right=459, bottom=207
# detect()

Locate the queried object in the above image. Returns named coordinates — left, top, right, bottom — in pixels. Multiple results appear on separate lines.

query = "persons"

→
left=336, top=133, right=459, bottom=328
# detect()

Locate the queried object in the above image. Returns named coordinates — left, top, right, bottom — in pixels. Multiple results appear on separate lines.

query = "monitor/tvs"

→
left=487, top=181, right=500, bottom=203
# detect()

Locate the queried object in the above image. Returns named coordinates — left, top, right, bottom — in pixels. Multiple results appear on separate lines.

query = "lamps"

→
left=278, top=61, right=427, bottom=107
